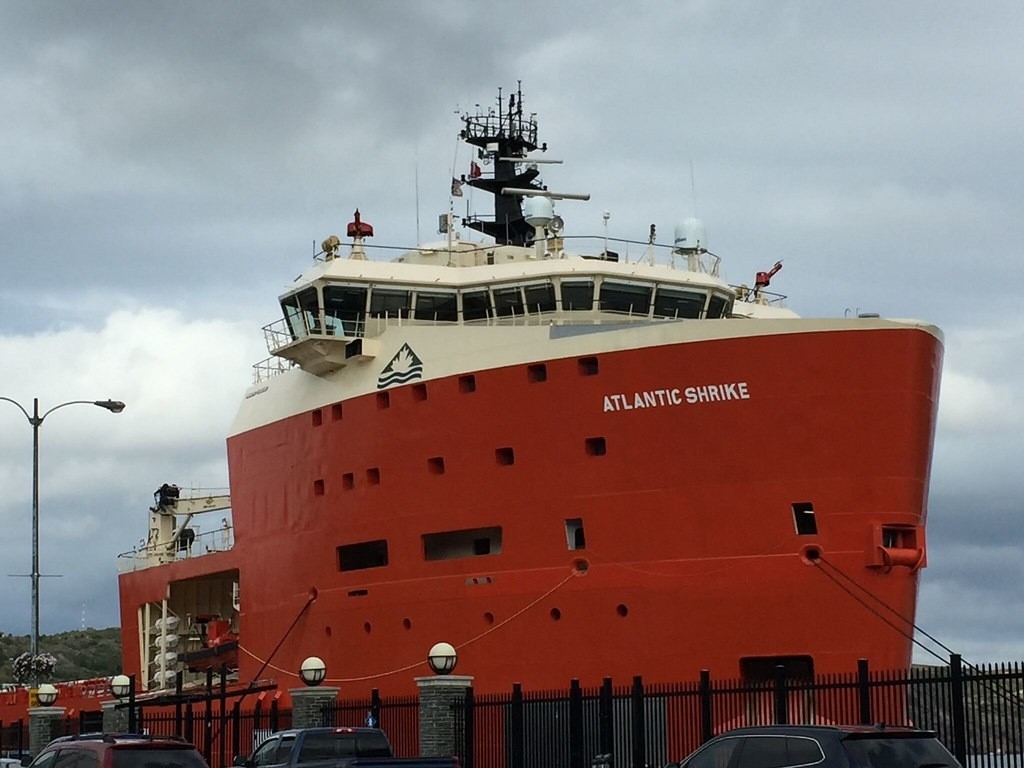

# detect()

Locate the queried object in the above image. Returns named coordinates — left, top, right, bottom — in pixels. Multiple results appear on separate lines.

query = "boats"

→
left=105, top=72, right=948, bottom=768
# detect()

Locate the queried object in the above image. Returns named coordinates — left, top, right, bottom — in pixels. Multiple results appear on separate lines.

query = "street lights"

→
left=0, top=398, right=127, bottom=673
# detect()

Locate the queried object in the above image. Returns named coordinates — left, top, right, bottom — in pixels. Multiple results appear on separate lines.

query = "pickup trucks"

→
left=229, top=726, right=463, bottom=768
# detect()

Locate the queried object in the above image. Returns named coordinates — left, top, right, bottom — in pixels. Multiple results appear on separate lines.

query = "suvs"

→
left=659, top=722, right=969, bottom=767
left=20, top=729, right=211, bottom=768
